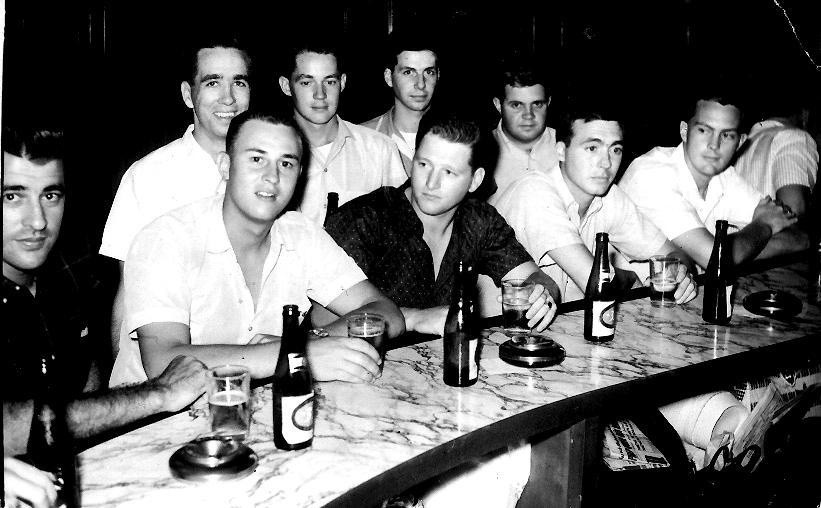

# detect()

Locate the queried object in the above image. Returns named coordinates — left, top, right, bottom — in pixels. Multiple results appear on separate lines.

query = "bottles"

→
left=443, top=258, right=483, bottom=388
left=272, top=304, right=315, bottom=451
left=323, top=192, right=339, bottom=229
left=701, top=219, right=738, bottom=325
left=583, top=232, right=616, bottom=343
left=25, top=352, right=82, bottom=508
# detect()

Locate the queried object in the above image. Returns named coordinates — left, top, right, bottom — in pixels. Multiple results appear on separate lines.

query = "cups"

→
left=502, top=280, right=536, bottom=337
left=650, top=255, right=679, bottom=308
left=205, top=365, right=251, bottom=443
left=348, top=313, right=385, bottom=376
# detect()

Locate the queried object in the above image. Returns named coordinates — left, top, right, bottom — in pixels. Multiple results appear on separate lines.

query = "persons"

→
left=324, top=107, right=559, bottom=339
left=618, top=90, right=810, bottom=268
left=4, top=456, right=60, bottom=508
left=494, top=102, right=699, bottom=305
left=109, top=104, right=406, bottom=389
left=735, top=81, right=820, bottom=227
left=482, top=69, right=559, bottom=207
left=357, top=29, right=440, bottom=179
left=1, top=119, right=217, bottom=457
left=277, top=43, right=410, bottom=230
left=99, top=35, right=250, bottom=359
